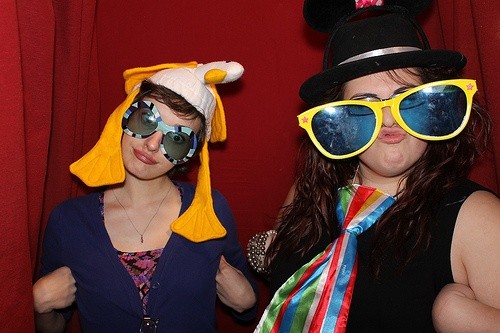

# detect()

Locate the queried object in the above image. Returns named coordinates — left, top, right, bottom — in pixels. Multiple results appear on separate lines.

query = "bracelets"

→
left=247, top=230, right=277, bottom=276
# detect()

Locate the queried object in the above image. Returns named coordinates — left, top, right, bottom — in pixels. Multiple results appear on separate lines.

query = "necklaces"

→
left=352, top=162, right=407, bottom=198
left=111, top=183, right=172, bottom=243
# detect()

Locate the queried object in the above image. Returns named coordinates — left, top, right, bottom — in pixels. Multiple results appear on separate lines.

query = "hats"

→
left=298, top=0, right=467, bottom=108
left=69, top=61, right=245, bottom=242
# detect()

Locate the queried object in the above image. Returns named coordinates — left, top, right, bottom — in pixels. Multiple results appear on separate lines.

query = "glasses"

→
left=297, top=78, right=479, bottom=160
left=121, top=100, right=196, bottom=164
left=340, top=90, right=433, bottom=116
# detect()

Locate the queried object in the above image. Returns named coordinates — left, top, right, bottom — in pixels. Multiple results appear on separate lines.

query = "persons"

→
left=32, top=61, right=259, bottom=333
left=246, top=0, right=500, bottom=333
